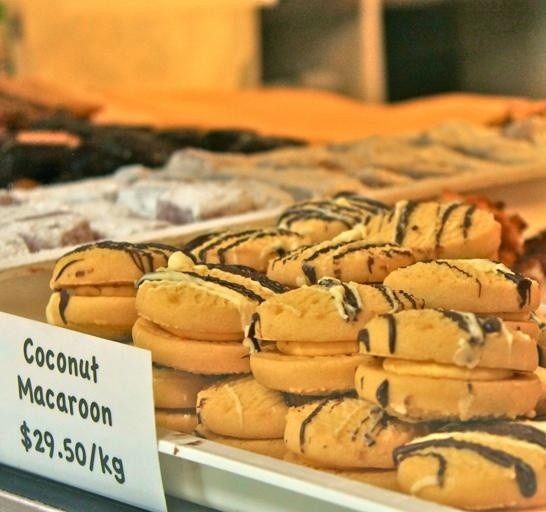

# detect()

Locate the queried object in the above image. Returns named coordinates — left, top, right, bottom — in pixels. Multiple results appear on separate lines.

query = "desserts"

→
left=0, top=110, right=546, bottom=512
